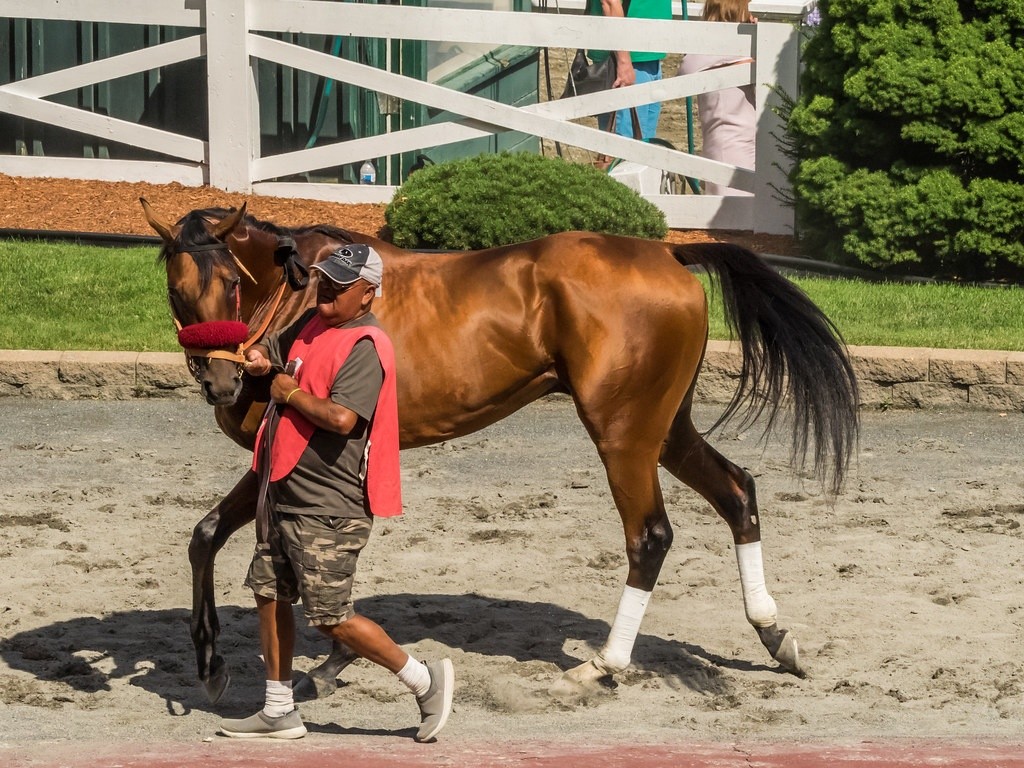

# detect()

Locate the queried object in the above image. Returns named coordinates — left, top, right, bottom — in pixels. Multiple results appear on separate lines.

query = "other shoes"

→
left=219, top=705, right=307, bottom=739
left=416, top=658, right=455, bottom=741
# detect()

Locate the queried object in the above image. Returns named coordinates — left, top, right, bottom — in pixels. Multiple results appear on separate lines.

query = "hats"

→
left=309, top=243, right=384, bottom=288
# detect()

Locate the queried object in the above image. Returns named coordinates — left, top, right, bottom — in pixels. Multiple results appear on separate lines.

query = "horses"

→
left=139, top=197, right=860, bottom=705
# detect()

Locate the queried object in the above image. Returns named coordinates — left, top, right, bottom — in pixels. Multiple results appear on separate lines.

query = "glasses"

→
left=321, top=272, right=370, bottom=291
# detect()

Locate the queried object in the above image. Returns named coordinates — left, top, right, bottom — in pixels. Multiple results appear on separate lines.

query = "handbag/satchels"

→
left=559, top=51, right=617, bottom=118
left=590, top=108, right=642, bottom=173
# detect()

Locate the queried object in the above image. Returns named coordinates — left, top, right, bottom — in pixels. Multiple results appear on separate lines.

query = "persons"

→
left=215, top=241, right=457, bottom=744
left=585, top=1, right=674, bottom=169
left=680, top=0, right=754, bottom=195
